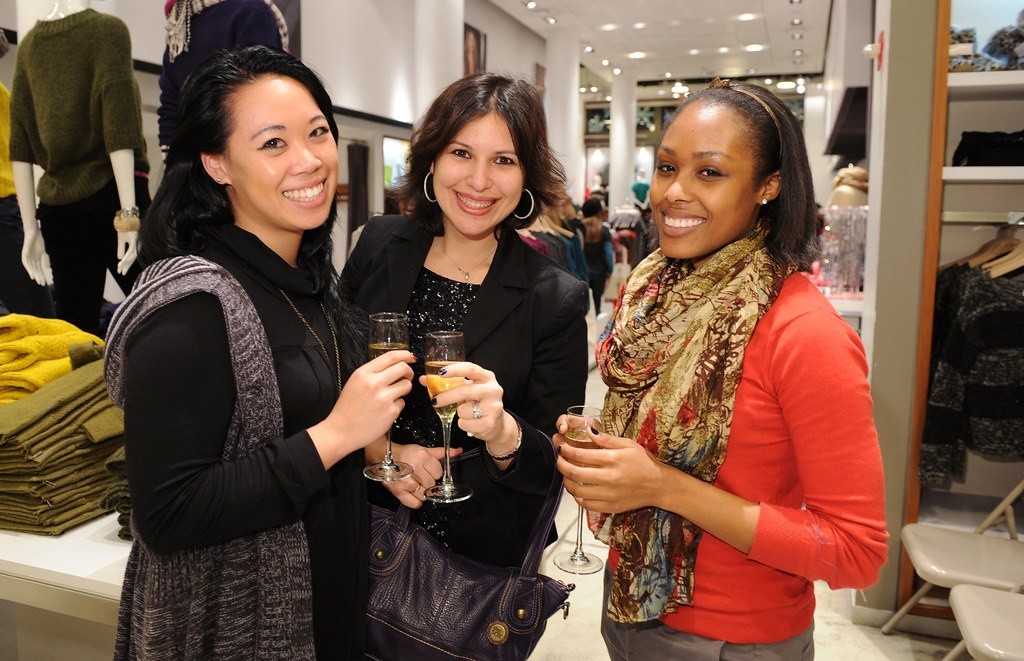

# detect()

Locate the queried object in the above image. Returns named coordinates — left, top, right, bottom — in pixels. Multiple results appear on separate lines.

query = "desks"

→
left=0, top=505, right=136, bottom=661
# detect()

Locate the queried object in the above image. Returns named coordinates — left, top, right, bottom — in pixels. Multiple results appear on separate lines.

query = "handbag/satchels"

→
left=367, top=428, right=576, bottom=661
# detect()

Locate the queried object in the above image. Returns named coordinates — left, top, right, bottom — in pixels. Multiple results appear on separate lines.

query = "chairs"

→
left=881, top=477, right=1024, bottom=661
left=940, top=585, right=1024, bottom=661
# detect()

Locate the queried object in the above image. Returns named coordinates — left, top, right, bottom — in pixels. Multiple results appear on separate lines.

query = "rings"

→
left=410, top=485, right=421, bottom=493
left=473, top=400, right=481, bottom=419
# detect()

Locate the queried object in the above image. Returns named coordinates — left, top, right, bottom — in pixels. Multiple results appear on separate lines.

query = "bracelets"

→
left=486, top=417, right=522, bottom=461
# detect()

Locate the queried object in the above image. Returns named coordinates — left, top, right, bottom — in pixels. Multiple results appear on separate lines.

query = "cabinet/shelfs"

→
left=897, top=0, right=1024, bottom=622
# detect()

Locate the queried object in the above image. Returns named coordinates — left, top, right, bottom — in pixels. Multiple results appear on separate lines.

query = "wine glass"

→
left=364, top=312, right=413, bottom=483
left=552, top=406, right=608, bottom=575
left=422, top=330, right=473, bottom=504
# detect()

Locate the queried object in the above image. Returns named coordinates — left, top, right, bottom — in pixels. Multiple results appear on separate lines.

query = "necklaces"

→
left=279, top=286, right=341, bottom=396
left=442, top=243, right=494, bottom=280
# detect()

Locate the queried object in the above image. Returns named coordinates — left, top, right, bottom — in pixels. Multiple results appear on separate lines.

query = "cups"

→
left=818, top=206, right=867, bottom=294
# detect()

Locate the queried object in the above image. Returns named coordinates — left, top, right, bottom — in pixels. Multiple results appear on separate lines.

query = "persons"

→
left=335, top=72, right=590, bottom=567
left=104, top=46, right=417, bottom=661
left=553, top=79, right=889, bottom=661
left=575, top=200, right=615, bottom=319
left=0, top=1, right=290, bottom=335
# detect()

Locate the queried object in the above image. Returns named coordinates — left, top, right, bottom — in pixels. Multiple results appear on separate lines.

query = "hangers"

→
left=940, top=224, right=1024, bottom=279
